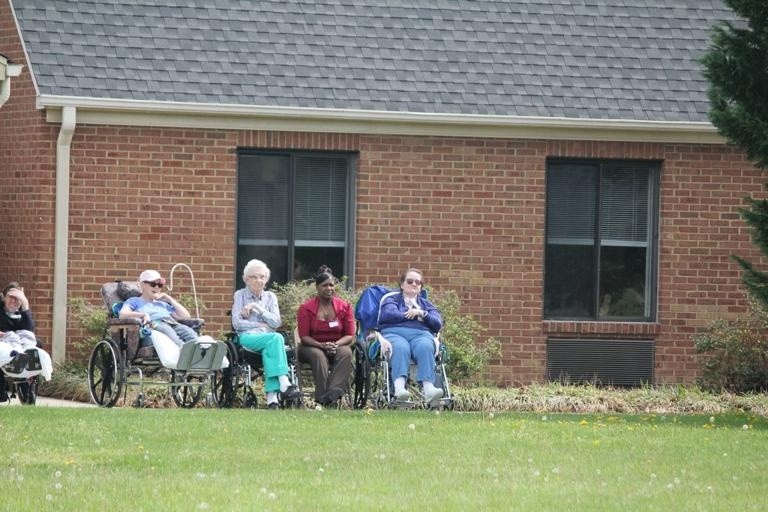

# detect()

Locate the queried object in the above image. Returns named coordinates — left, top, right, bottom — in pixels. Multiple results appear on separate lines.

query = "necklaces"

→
left=319, top=301, right=332, bottom=317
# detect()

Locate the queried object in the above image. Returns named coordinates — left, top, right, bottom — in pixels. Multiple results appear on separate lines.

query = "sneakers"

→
left=394, top=389, right=411, bottom=402
left=424, top=387, right=444, bottom=404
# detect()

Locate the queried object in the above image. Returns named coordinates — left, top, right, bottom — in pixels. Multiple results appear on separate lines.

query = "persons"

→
left=116, top=269, right=217, bottom=370
left=230, top=258, right=304, bottom=410
left=295, top=263, right=357, bottom=411
left=375, top=267, right=445, bottom=404
left=0, top=282, right=41, bottom=375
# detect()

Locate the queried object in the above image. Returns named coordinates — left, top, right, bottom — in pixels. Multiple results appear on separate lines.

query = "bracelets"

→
left=140, top=312, right=148, bottom=319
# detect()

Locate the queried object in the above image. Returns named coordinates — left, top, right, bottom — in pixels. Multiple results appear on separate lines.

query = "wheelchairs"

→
left=354, top=286, right=456, bottom=412
left=0, top=292, right=52, bottom=406
left=88, top=279, right=226, bottom=408
left=292, top=320, right=372, bottom=410
left=210, top=309, right=301, bottom=410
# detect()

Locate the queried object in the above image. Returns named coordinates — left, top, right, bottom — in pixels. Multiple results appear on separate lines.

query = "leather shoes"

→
left=267, top=402, right=287, bottom=410
left=324, top=400, right=342, bottom=410
left=279, top=384, right=303, bottom=400
left=317, top=387, right=345, bottom=404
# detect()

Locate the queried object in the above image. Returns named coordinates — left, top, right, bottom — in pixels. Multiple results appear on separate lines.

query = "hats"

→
left=139, top=269, right=166, bottom=285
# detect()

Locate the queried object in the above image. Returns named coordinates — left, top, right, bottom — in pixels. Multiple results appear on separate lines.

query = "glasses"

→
left=404, top=278, right=422, bottom=286
left=247, top=275, right=266, bottom=280
left=143, top=281, right=163, bottom=288
